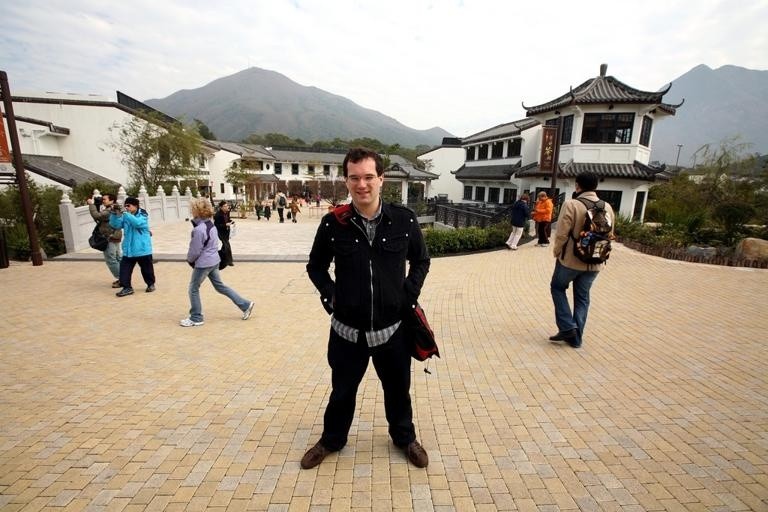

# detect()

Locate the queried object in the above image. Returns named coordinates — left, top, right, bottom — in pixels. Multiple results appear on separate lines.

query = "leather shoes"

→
left=299, top=444, right=330, bottom=470
left=549, top=329, right=577, bottom=341
left=404, top=440, right=429, bottom=468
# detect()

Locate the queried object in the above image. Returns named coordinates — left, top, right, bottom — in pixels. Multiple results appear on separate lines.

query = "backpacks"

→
left=572, top=198, right=616, bottom=266
left=278, top=194, right=286, bottom=206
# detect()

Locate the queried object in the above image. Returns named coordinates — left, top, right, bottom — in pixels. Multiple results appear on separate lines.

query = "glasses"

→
left=345, top=175, right=381, bottom=185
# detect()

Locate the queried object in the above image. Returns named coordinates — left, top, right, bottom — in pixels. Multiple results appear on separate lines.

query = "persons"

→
left=213, top=201, right=236, bottom=269
left=178, top=198, right=255, bottom=327
left=505, top=194, right=531, bottom=251
left=86, top=193, right=124, bottom=288
left=297, top=148, right=431, bottom=469
left=549, top=171, right=615, bottom=349
left=255, top=188, right=321, bottom=224
left=109, top=197, right=156, bottom=297
left=529, top=191, right=554, bottom=247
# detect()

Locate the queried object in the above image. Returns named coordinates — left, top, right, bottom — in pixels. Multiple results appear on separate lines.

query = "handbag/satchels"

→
left=88, top=226, right=109, bottom=251
left=407, top=303, right=441, bottom=362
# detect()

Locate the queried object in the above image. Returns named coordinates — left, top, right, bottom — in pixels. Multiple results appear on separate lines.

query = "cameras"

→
left=87, top=197, right=103, bottom=205
left=114, top=205, right=122, bottom=210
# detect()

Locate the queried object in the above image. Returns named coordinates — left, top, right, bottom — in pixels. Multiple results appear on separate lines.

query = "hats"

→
left=124, top=197, right=139, bottom=207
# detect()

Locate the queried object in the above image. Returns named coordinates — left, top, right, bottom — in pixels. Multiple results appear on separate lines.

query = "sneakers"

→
left=241, top=301, right=254, bottom=321
left=116, top=286, right=134, bottom=297
left=146, top=284, right=155, bottom=292
left=112, top=280, right=121, bottom=288
left=179, top=317, right=204, bottom=327
